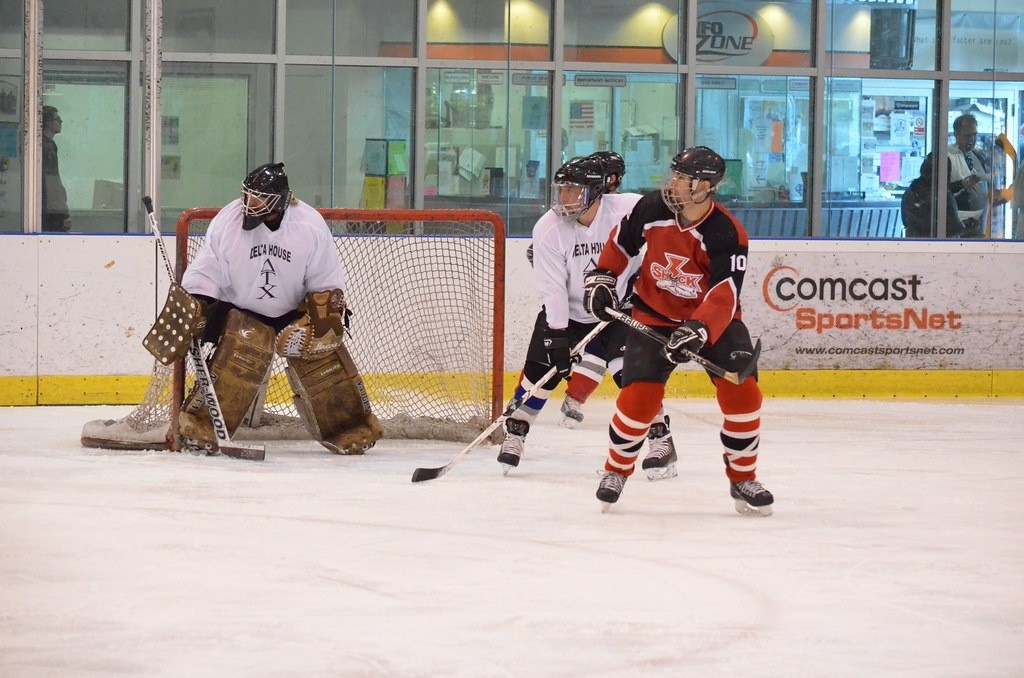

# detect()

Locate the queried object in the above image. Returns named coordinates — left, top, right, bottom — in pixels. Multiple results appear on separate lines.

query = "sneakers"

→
left=730, top=480, right=773, bottom=517
left=596, top=469, right=628, bottom=513
left=557, top=395, right=584, bottom=429
left=497, top=403, right=530, bottom=475
left=642, top=416, right=678, bottom=481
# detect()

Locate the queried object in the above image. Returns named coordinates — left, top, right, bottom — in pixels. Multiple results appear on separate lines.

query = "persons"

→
left=497, top=151, right=679, bottom=480
left=900, top=151, right=965, bottom=238
left=946, top=113, right=1006, bottom=238
left=583, top=145, right=774, bottom=517
left=166, top=162, right=385, bottom=455
left=42, top=106, right=72, bottom=232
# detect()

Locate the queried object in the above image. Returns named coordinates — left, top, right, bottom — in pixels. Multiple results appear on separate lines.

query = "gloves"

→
left=664, top=320, right=708, bottom=364
left=584, top=268, right=619, bottom=322
left=543, top=326, right=581, bottom=380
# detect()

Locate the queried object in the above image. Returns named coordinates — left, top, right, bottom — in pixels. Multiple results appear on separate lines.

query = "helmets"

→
left=588, top=151, right=625, bottom=191
left=241, top=162, right=292, bottom=232
left=551, top=158, right=605, bottom=222
left=662, top=146, right=726, bottom=213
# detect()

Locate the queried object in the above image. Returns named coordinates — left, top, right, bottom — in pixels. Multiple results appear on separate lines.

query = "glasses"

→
left=964, top=155, right=973, bottom=171
left=45, top=117, right=61, bottom=120
